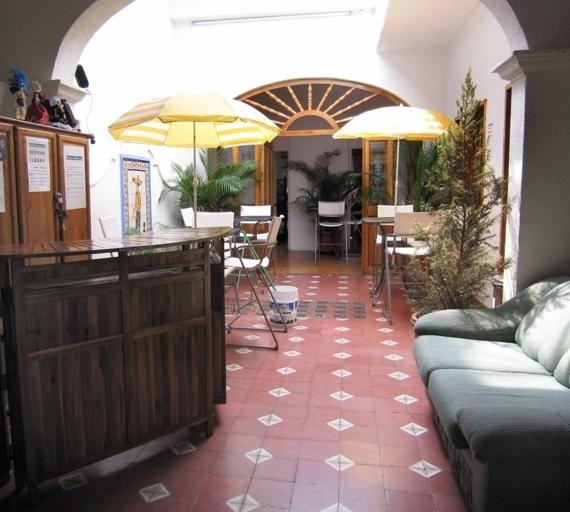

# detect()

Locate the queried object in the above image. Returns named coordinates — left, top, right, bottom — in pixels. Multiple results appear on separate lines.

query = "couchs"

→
left=411, top=269, right=570, bottom=512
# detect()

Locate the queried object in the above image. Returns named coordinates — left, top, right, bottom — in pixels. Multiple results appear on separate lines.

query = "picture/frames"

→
left=121, top=155, right=152, bottom=237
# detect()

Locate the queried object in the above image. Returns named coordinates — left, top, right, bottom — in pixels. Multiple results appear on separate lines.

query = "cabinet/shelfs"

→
left=0, top=116, right=94, bottom=268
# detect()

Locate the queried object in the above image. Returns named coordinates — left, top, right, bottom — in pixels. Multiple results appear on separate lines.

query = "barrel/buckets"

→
left=270, top=284, right=299, bottom=325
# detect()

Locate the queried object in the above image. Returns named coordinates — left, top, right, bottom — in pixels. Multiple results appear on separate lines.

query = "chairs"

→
left=315, top=199, right=459, bottom=323
left=178, top=206, right=287, bottom=351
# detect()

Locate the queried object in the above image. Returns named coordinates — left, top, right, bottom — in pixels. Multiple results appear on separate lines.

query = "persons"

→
left=132, top=182, right=143, bottom=233
left=8, top=66, right=82, bottom=131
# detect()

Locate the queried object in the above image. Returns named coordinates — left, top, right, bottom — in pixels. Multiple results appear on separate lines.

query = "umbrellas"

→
left=333, top=103, right=456, bottom=212
left=109, top=92, right=283, bottom=228
